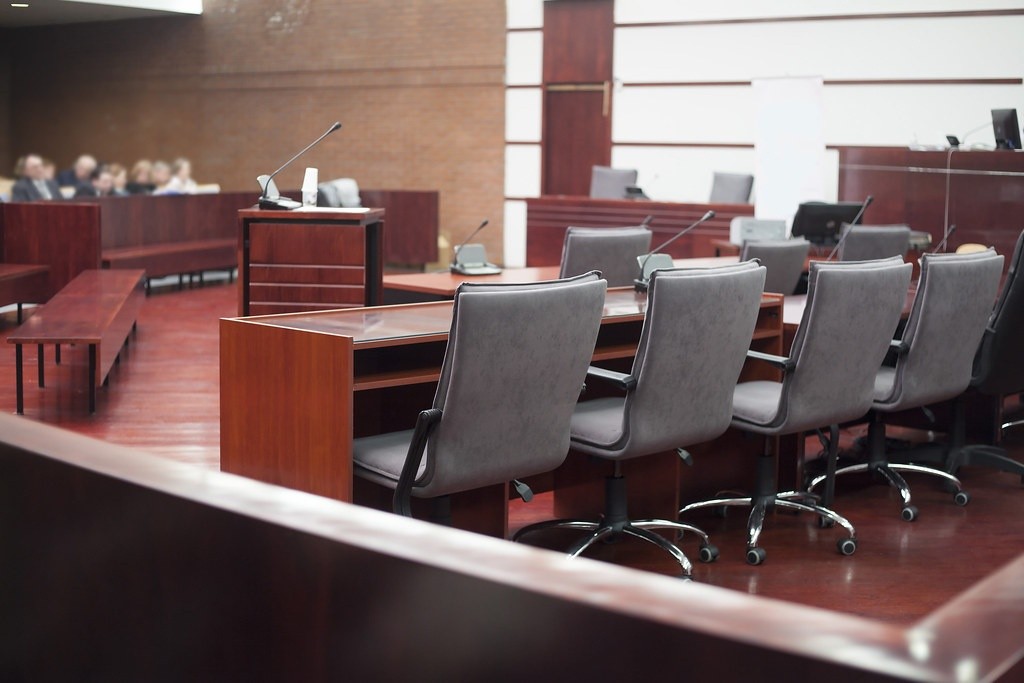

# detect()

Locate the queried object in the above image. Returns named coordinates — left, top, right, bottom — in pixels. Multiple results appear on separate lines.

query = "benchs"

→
left=100, top=237, right=239, bottom=295
left=7, top=268, right=149, bottom=414
left=0, top=262, right=51, bottom=327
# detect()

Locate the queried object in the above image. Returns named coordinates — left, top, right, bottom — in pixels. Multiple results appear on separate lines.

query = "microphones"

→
left=258, top=122, right=342, bottom=210
left=450, top=220, right=501, bottom=276
left=634, top=210, right=715, bottom=291
left=932, top=225, right=956, bottom=254
left=825, top=195, right=873, bottom=262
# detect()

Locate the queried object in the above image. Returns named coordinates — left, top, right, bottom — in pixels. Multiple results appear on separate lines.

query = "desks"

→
left=525, top=196, right=754, bottom=267
left=219, top=286, right=787, bottom=544
left=382, top=265, right=563, bottom=305
left=827, top=144, right=1024, bottom=282
left=783, top=279, right=1004, bottom=513
left=713, top=240, right=839, bottom=274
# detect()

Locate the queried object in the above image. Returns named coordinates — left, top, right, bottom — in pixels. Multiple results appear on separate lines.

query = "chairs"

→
left=354, top=161, right=1024, bottom=582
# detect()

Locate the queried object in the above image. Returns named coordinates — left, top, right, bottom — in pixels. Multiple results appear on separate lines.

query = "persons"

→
left=0, top=153, right=198, bottom=203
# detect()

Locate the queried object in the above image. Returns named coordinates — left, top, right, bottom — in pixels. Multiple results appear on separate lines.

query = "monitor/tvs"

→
left=991, top=108, right=1022, bottom=151
left=798, top=203, right=863, bottom=249
